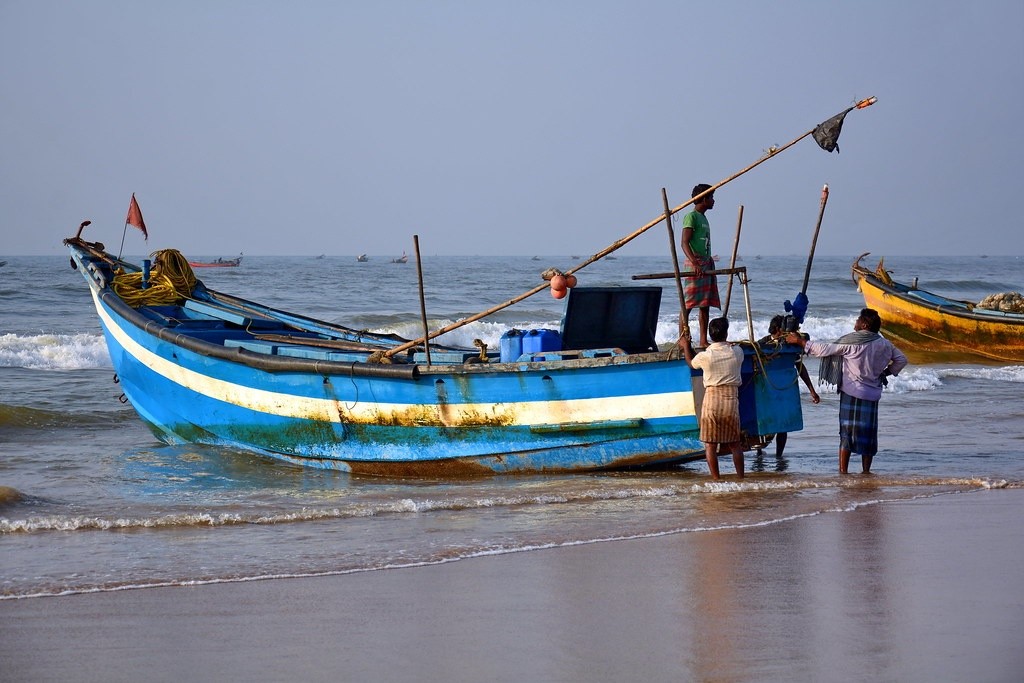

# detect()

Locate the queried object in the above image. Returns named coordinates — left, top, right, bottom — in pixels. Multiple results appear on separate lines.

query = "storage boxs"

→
left=909, top=291, right=955, bottom=307
left=141, top=306, right=225, bottom=330
left=224, top=339, right=501, bottom=365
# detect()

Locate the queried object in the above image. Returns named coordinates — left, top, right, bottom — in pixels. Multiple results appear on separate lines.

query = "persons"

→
left=786, top=301, right=908, bottom=475
left=754, top=313, right=820, bottom=460
left=678, top=317, right=747, bottom=483
left=679, top=183, right=721, bottom=346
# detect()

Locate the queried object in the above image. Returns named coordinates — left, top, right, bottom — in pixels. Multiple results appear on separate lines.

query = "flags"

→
left=126, top=197, right=149, bottom=241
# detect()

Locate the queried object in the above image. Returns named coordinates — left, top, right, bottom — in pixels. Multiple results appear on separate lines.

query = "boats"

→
left=392, top=251, right=407, bottom=263
left=755, top=254, right=762, bottom=259
left=604, top=256, right=617, bottom=261
left=186, top=252, right=245, bottom=267
left=729, top=253, right=744, bottom=262
left=849, top=252, right=1024, bottom=367
left=0, top=261, right=9, bottom=268
left=570, top=255, right=581, bottom=261
left=62, top=183, right=831, bottom=485
left=357, top=254, right=369, bottom=263
left=710, top=254, right=719, bottom=262
left=531, top=256, right=541, bottom=262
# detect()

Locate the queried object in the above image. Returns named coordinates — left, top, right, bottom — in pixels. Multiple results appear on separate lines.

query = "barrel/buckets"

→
left=500, top=329, right=527, bottom=363
left=522, top=329, right=558, bottom=362
